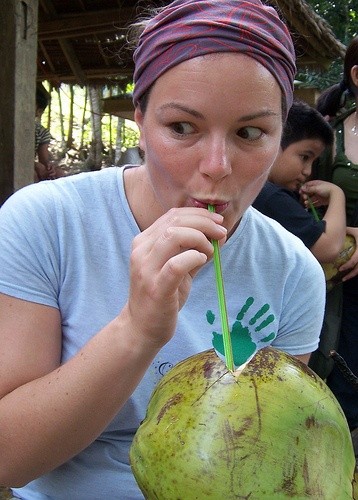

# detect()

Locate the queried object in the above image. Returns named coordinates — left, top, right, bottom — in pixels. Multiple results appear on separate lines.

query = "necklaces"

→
left=352, top=110, right=358, bottom=135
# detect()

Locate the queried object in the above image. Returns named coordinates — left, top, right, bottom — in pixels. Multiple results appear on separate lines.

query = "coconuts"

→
left=129, top=346, right=358, bottom=500
left=320, top=235, right=356, bottom=284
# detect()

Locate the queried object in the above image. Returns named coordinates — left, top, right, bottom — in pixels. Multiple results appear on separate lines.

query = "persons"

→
left=34, top=82, right=64, bottom=182
left=252, top=101, right=347, bottom=261
left=0, top=0, right=326, bottom=500
left=308, top=41, right=358, bottom=460
